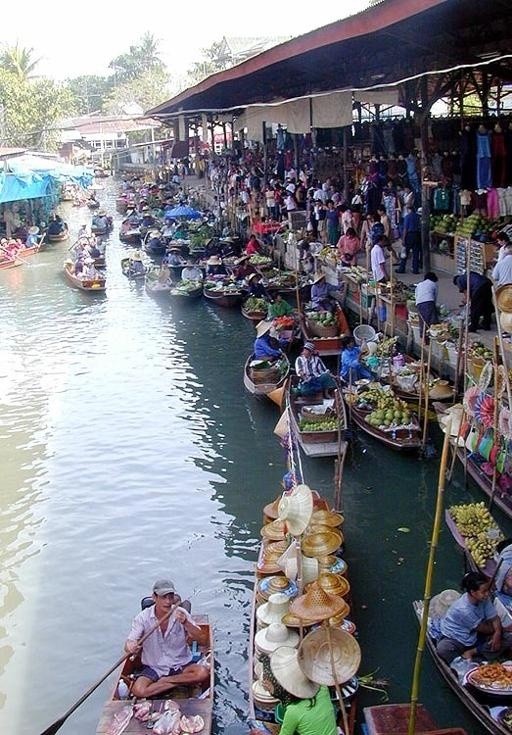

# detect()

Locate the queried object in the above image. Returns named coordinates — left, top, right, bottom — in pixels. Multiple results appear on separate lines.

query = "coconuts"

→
left=365, top=408, right=410, bottom=425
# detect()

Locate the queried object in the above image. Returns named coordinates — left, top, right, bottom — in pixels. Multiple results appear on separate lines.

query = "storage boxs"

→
left=283, top=223, right=501, bottom=389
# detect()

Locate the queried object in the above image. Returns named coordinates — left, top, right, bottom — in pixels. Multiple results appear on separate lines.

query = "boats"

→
left=362, top=704, right=468, bottom=734
left=430, top=400, right=512, bottom=521
left=412, top=595, right=511, bottom=734
left=46, top=223, right=69, bottom=242
left=348, top=324, right=459, bottom=408
left=95, top=613, right=215, bottom=735
left=443, top=502, right=512, bottom=626
left=265, top=373, right=348, bottom=460
left=59, top=164, right=353, bottom=411
left=249, top=490, right=358, bottom=735
left=62, top=259, right=107, bottom=291
left=1, top=253, right=23, bottom=272
left=19, top=242, right=46, bottom=256
left=343, top=379, right=423, bottom=450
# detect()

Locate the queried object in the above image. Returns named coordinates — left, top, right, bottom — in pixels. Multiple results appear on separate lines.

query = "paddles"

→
left=41, top=600, right=182, bottom=735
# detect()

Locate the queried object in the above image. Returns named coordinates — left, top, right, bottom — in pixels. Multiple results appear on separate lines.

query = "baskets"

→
left=309, top=321, right=339, bottom=337
left=301, top=407, right=333, bottom=423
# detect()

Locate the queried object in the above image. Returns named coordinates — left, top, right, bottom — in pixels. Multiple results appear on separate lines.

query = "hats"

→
left=312, top=272, right=326, bottom=285
left=256, top=580, right=361, bottom=700
left=304, top=342, right=315, bottom=352
left=254, top=320, right=273, bottom=338
left=499, top=313, right=512, bottom=334
left=257, top=531, right=350, bottom=599
left=491, top=283, right=512, bottom=313
left=29, top=226, right=39, bottom=234
left=429, top=380, right=453, bottom=397
left=207, top=256, right=220, bottom=265
left=259, top=484, right=344, bottom=540
left=463, top=361, right=512, bottom=440
left=153, top=579, right=176, bottom=596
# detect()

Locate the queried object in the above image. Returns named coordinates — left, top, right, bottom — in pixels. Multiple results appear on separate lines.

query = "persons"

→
left=125, top=579, right=210, bottom=698
left=452, top=271, right=494, bottom=333
left=437, top=572, right=512, bottom=667
left=493, top=543, right=512, bottom=634
left=1, top=215, right=64, bottom=260
left=492, top=242, right=512, bottom=290
left=278, top=681, right=338, bottom=735
left=75, top=194, right=112, bottom=281
left=236, top=320, right=372, bottom=391
left=122, top=152, right=234, bottom=285
left=497, top=231, right=510, bottom=261
left=207, top=151, right=438, bottom=346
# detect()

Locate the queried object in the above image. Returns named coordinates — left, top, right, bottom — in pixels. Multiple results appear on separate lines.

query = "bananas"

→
left=449, top=501, right=505, bottom=570
